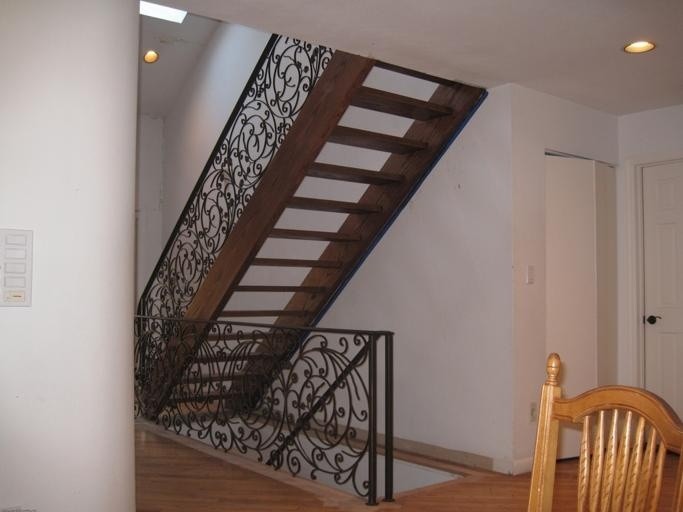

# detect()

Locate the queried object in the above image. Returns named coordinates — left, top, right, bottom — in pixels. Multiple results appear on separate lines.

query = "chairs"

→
left=525, top=353, right=681, bottom=511
